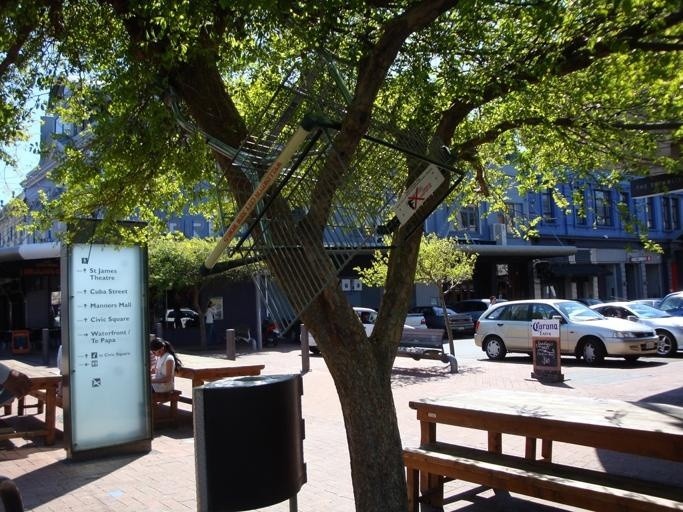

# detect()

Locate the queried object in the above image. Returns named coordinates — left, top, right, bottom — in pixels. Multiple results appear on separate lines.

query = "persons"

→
left=150, top=337, right=182, bottom=393
left=488, top=296, right=496, bottom=308
left=204, top=301, right=216, bottom=346
left=499, top=285, right=503, bottom=299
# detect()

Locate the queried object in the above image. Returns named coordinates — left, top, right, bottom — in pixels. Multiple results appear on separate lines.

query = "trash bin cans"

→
left=192, top=374, right=308, bottom=512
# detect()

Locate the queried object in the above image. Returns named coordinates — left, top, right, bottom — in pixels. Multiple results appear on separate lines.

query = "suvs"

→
left=451, top=299, right=510, bottom=329
left=165, top=307, right=199, bottom=328
left=654, top=291, right=683, bottom=317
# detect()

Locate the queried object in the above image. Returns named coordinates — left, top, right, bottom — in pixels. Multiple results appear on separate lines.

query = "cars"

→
left=299, top=305, right=415, bottom=354
left=572, top=297, right=603, bottom=307
left=630, top=298, right=659, bottom=309
left=409, top=306, right=473, bottom=338
left=404, top=311, right=428, bottom=331
left=474, top=298, right=659, bottom=365
left=569, top=302, right=683, bottom=356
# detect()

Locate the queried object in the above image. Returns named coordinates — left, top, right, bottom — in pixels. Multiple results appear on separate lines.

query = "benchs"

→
left=16, top=387, right=67, bottom=423
left=400, top=438, right=683, bottom=511
left=393, top=327, right=457, bottom=375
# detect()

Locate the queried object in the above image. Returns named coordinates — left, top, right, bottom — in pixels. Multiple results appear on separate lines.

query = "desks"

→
left=409, top=388, right=682, bottom=511
left=152, top=352, right=264, bottom=415
left=0, top=355, right=60, bottom=452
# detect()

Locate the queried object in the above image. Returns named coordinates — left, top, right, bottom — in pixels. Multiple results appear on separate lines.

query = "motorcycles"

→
left=261, top=315, right=280, bottom=349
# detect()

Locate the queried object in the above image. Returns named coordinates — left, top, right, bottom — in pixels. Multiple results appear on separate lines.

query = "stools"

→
left=149, top=388, right=181, bottom=430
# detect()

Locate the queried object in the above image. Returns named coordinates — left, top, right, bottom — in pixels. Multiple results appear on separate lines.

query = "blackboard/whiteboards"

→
left=532, top=336, right=560, bottom=371
